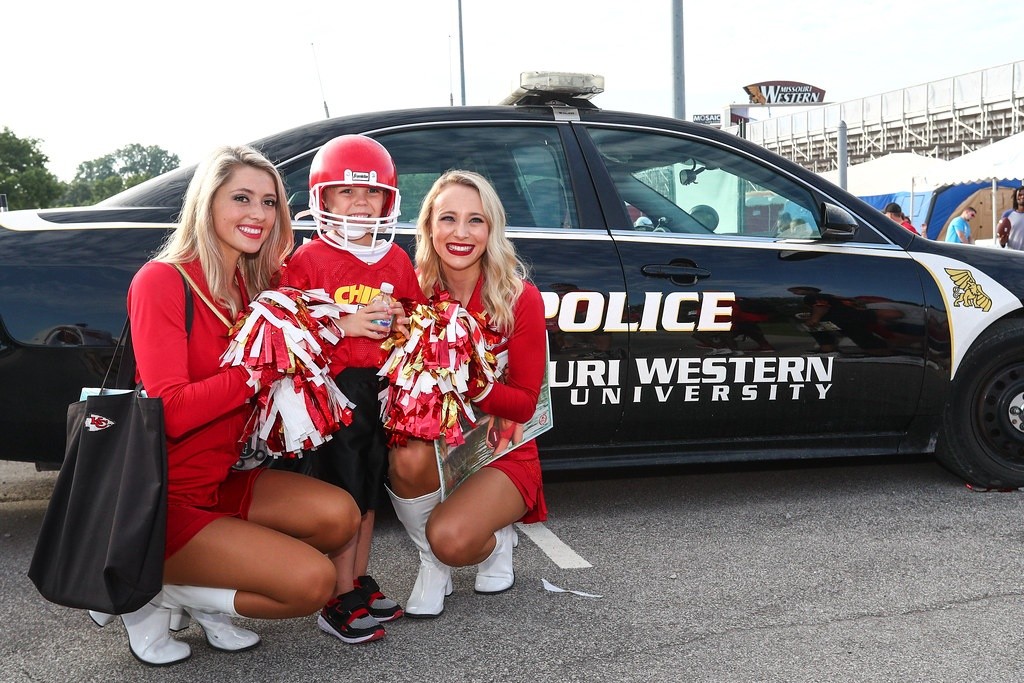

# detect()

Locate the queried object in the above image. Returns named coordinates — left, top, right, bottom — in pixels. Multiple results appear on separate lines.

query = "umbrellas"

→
left=814, top=131, right=1024, bottom=244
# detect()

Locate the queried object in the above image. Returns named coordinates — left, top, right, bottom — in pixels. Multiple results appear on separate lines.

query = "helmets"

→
left=308, top=134, right=397, bottom=240
left=633, top=216, right=655, bottom=229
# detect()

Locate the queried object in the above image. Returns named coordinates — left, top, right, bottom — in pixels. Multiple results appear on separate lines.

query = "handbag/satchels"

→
left=28, top=255, right=195, bottom=614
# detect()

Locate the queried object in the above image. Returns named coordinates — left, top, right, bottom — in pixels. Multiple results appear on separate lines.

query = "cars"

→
left=0, top=70, right=1024, bottom=491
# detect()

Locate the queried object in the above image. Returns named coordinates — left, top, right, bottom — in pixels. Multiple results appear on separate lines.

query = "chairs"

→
left=519, top=179, right=567, bottom=230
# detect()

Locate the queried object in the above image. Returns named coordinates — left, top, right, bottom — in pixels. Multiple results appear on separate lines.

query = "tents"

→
left=746, top=191, right=1024, bottom=237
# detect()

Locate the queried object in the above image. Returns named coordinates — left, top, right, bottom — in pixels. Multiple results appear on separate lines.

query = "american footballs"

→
left=995, top=216, right=1011, bottom=246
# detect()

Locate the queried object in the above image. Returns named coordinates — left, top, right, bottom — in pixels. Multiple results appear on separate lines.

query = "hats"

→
left=881, top=203, right=901, bottom=214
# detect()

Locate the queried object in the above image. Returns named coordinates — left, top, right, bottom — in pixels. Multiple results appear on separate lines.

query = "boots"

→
left=382, top=481, right=454, bottom=619
left=169, top=601, right=260, bottom=652
left=88, top=585, right=249, bottom=667
left=474, top=524, right=519, bottom=595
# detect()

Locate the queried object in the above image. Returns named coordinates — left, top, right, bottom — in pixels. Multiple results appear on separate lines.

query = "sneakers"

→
left=317, top=589, right=385, bottom=644
left=353, top=575, right=405, bottom=622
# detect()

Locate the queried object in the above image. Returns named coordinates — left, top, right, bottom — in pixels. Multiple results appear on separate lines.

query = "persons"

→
left=88, top=144, right=361, bottom=667
left=771, top=185, right=1024, bottom=251
left=383, top=170, right=547, bottom=616
left=278, top=135, right=431, bottom=643
